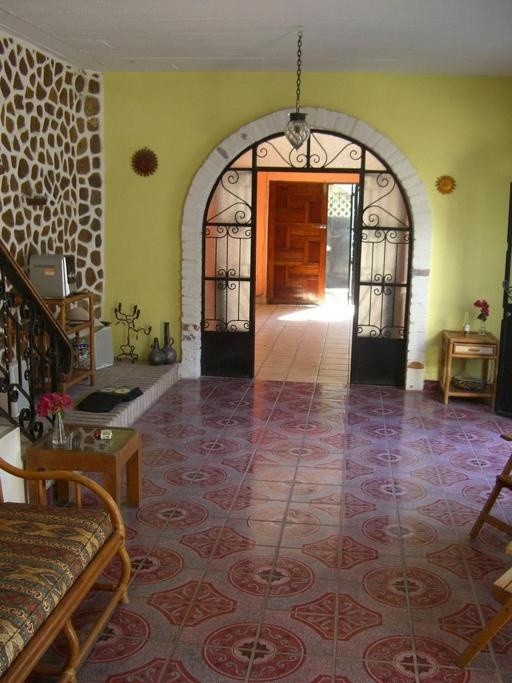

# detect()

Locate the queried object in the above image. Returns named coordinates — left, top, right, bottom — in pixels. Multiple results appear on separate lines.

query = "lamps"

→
left=285, top=26, right=311, bottom=153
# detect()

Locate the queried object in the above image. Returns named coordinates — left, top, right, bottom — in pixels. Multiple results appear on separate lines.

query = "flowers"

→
left=473, top=298, right=490, bottom=321
left=35, top=392, right=75, bottom=418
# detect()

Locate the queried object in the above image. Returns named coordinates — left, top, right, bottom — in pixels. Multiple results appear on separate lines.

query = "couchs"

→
left=1, top=463, right=133, bottom=683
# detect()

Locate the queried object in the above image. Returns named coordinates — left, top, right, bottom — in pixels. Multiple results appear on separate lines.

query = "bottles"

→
left=462, top=311, right=473, bottom=335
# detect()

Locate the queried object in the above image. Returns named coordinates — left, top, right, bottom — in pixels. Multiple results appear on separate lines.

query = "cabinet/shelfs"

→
left=444, top=343, right=500, bottom=406
left=29, top=292, right=97, bottom=393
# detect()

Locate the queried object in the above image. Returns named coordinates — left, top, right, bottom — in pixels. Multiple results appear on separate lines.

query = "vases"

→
left=463, top=311, right=471, bottom=335
left=479, top=321, right=487, bottom=336
left=51, top=412, right=68, bottom=446
